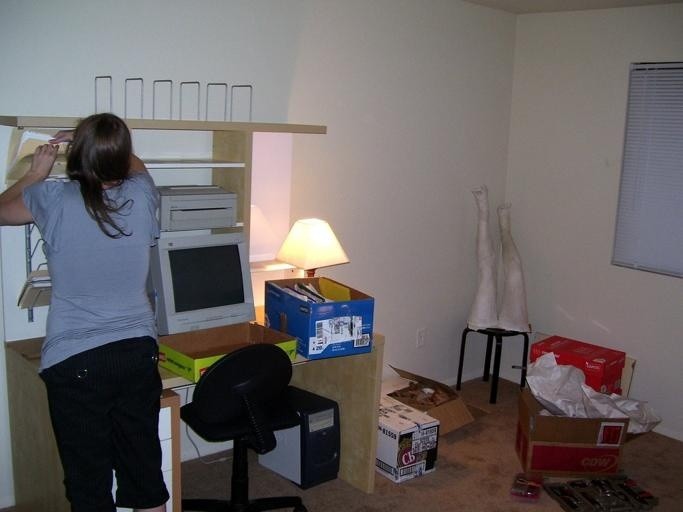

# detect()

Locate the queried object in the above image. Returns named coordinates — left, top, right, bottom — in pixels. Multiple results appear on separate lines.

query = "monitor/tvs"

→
left=146, top=227, right=256, bottom=336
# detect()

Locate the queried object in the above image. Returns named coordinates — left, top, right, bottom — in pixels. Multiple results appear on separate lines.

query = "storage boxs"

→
left=264, top=276, right=374, bottom=359
left=515, top=335, right=630, bottom=478
left=376, top=363, right=476, bottom=483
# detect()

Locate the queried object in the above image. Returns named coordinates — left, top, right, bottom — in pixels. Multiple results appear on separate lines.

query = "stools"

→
left=456, top=329, right=529, bottom=404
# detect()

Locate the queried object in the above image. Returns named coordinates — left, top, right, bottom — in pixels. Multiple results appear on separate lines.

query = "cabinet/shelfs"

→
left=110, top=395, right=181, bottom=511
left=17, top=158, right=245, bottom=310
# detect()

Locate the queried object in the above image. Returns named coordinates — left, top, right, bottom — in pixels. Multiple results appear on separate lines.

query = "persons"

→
left=1, top=112, right=172, bottom=512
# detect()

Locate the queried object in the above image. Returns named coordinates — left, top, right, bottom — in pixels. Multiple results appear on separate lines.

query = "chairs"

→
left=180, top=344, right=308, bottom=512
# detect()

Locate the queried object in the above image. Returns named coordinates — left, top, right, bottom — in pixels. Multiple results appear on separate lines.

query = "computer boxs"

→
left=258, top=385, right=341, bottom=490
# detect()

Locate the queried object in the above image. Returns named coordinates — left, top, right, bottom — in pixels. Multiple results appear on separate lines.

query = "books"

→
left=5, top=126, right=72, bottom=186
left=16, top=269, right=52, bottom=307
left=18, top=281, right=52, bottom=310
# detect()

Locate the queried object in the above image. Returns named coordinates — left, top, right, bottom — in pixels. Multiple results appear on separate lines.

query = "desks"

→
left=5, top=305, right=385, bottom=512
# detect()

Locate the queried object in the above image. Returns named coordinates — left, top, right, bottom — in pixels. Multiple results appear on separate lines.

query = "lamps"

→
left=275, top=218, right=351, bottom=276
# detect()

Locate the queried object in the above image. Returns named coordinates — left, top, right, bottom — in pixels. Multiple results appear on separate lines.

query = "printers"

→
left=156, top=185, right=238, bottom=232
